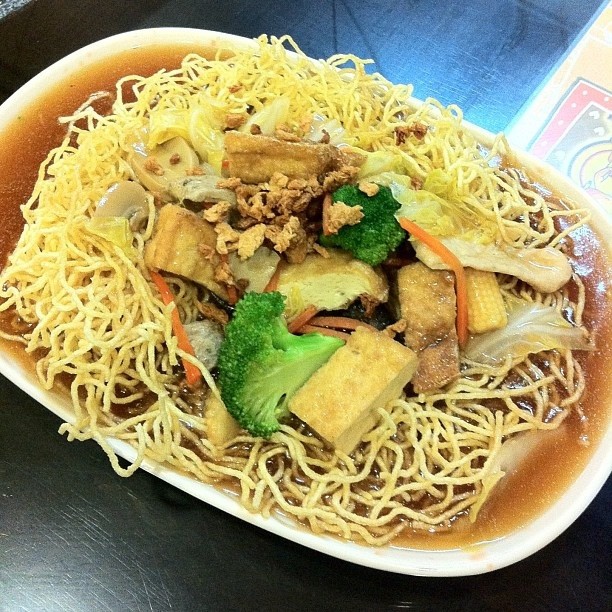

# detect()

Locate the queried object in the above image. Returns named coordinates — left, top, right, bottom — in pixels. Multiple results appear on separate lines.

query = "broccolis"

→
left=218, top=290, right=347, bottom=439
left=320, top=182, right=406, bottom=265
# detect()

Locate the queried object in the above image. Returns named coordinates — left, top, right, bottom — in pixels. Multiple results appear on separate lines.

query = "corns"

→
left=465, top=267, right=508, bottom=333
